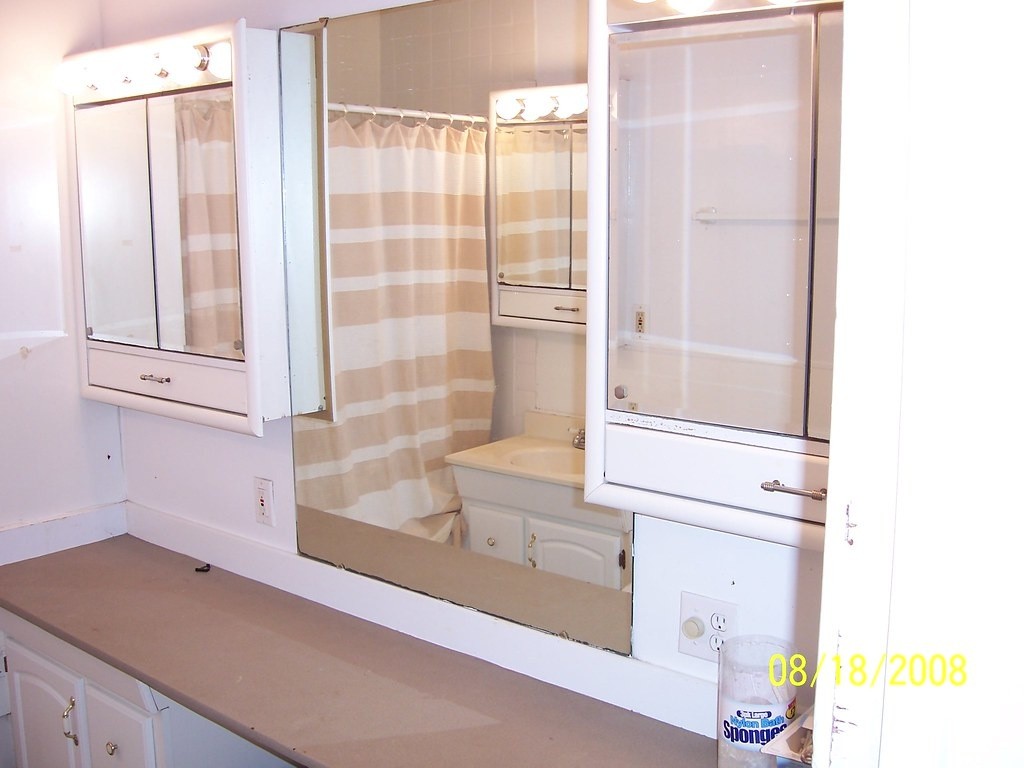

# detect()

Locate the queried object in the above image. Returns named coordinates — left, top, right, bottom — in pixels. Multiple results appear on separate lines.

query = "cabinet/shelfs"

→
left=583, top=0, right=1023, bottom=768
left=63, top=19, right=327, bottom=439
left=1, top=611, right=307, bottom=768
left=445, top=466, right=631, bottom=589
left=491, top=85, right=589, bottom=334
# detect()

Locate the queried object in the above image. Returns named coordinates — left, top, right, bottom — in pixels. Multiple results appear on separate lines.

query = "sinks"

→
left=509, top=450, right=585, bottom=475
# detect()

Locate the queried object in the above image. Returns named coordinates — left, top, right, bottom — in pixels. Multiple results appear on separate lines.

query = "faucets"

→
left=573, top=429, right=585, bottom=450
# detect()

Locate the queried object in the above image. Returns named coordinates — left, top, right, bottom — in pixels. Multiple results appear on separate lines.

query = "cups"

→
left=716, top=637, right=795, bottom=768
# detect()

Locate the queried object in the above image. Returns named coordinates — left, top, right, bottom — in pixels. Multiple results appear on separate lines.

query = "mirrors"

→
left=277, top=0, right=634, bottom=657
left=607, top=12, right=844, bottom=442
left=74, top=86, right=244, bottom=362
left=497, top=123, right=587, bottom=291
